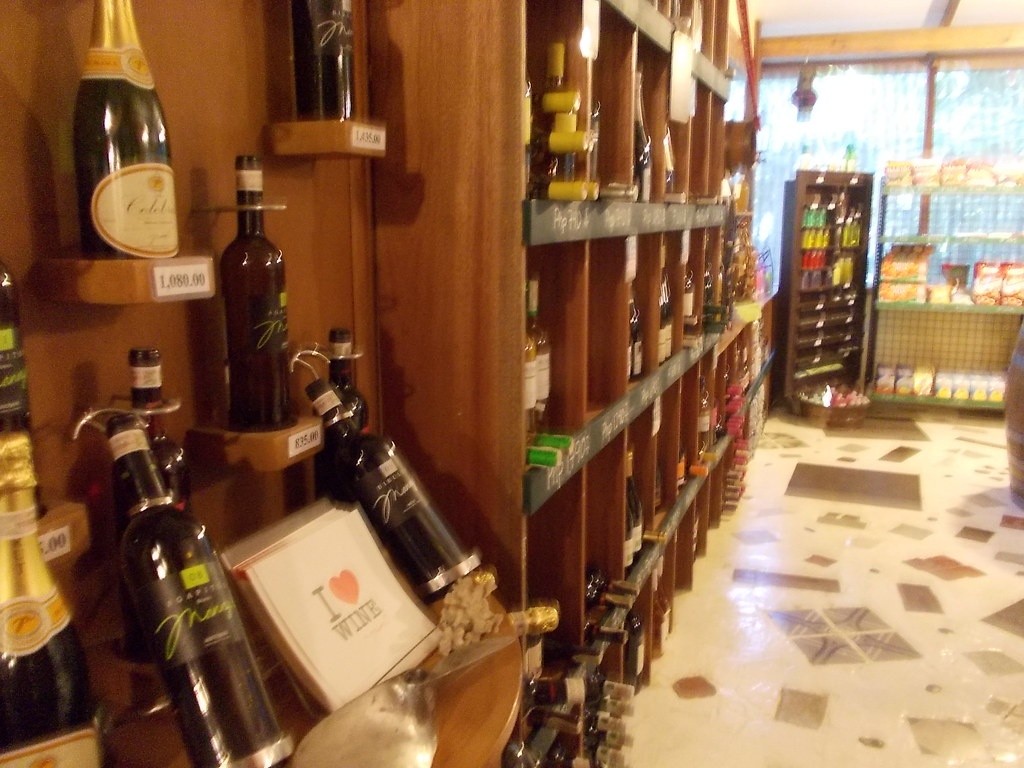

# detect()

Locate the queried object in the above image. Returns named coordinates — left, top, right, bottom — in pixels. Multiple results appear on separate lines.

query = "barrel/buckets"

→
left=1004, top=321, right=1024, bottom=500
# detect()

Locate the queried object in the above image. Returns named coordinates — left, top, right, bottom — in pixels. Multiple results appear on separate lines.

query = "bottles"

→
left=0, top=435, right=105, bottom=767
left=306, top=380, right=483, bottom=601
left=292, top=0, right=355, bottom=124
left=108, top=413, right=291, bottom=768
left=0, top=261, right=31, bottom=435
left=71, top=0, right=181, bottom=261
left=219, top=156, right=298, bottom=435
left=524, top=0, right=760, bottom=768
left=802, top=201, right=863, bottom=293
left=115, top=346, right=186, bottom=662
left=800, top=384, right=872, bottom=406
left=316, top=327, right=371, bottom=501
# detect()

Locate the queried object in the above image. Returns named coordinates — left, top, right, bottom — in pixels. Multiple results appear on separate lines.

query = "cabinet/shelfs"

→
left=364, top=0, right=778, bottom=767
left=864, top=174, right=1024, bottom=410
left=777, top=169, right=873, bottom=431
